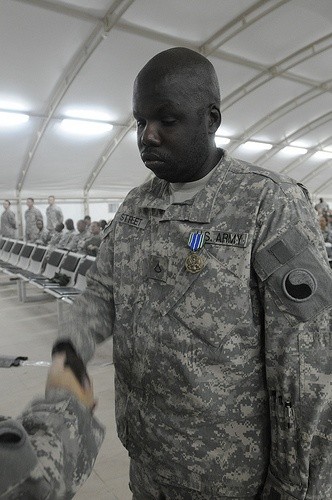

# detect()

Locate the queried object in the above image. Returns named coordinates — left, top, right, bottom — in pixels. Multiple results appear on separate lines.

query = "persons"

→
left=0, top=195, right=108, bottom=257
left=0, top=352, right=106, bottom=500
left=52, top=46, right=332, bottom=500
left=315, top=197, right=332, bottom=257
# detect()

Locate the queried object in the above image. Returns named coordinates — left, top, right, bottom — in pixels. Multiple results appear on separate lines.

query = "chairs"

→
left=0, top=237, right=98, bottom=321
